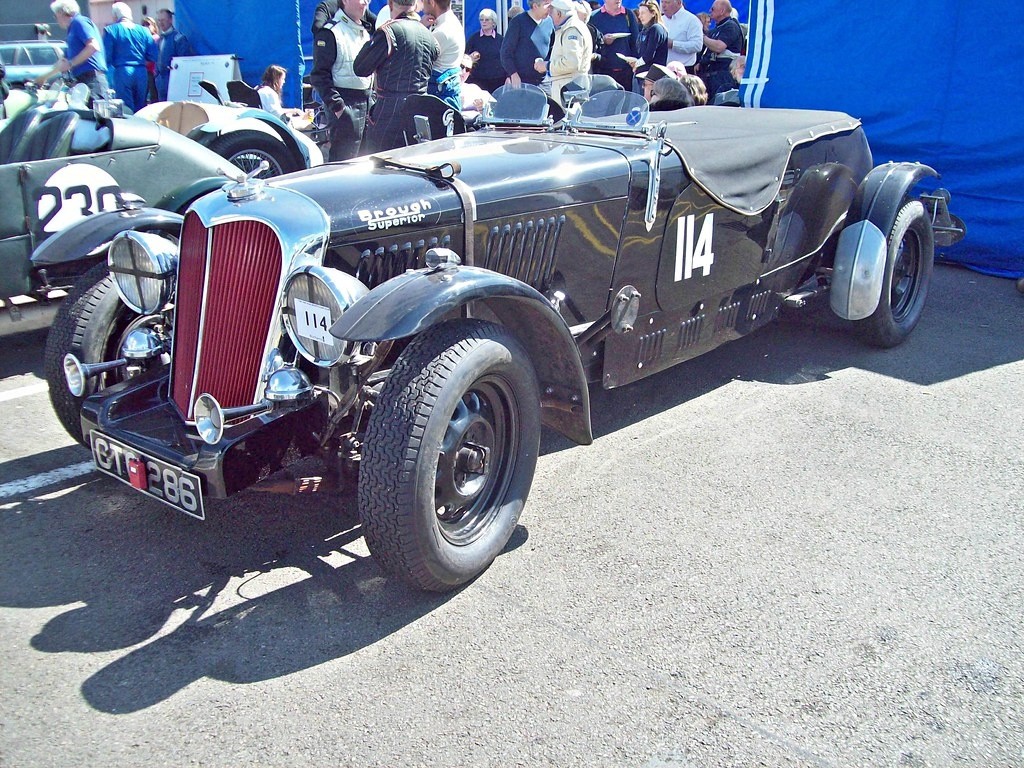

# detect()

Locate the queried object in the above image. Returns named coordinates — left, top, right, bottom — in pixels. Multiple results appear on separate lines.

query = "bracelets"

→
left=69, top=60, right=73, bottom=66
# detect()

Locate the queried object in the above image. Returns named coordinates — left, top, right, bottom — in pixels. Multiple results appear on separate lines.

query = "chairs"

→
left=302, top=76, right=324, bottom=138
left=400, top=92, right=467, bottom=144
left=225, top=79, right=262, bottom=108
left=196, top=79, right=226, bottom=104
left=559, top=73, right=626, bottom=116
left=0, top=107, right=43, bottom=164
left=493, top=89, right=565, bottom=122
left=11, top=110, right=81, bottom=163
left=714, top=88, right=739, bottom=106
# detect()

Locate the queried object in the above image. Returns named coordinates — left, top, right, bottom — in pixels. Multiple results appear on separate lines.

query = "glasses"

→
left=712, top=7, right=725, bottom=11
left=642, top=81, right=656, bottom=88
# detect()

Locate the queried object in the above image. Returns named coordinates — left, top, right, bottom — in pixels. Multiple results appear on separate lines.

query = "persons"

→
left=0, top=60, right=9, bottom=120
left=312, top=0, right=748, bottom=160
left=33, top=0, right=191, bottom=113
left=253, top=65, right=311, bottom=125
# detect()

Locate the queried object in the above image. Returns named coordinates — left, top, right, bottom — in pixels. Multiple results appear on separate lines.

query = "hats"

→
left=635, top=63, right=678, bottom=83
left=551, top=0, right=574, bottom=11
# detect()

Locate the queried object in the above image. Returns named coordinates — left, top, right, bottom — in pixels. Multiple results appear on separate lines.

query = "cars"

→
left=0, top=1, right=969, bottom=596
